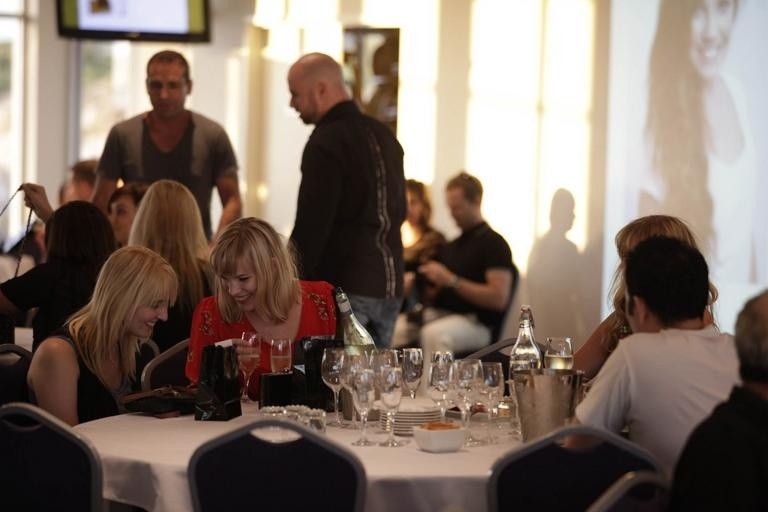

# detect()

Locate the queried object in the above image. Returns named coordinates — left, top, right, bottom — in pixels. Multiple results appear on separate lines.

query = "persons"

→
left=288, top=53, right=407, bottom=346
left=670, top=288, right=767, bottom=512
left=398, top=179, right=443, bottom=307
left=2, top=156, right=147, bottom=265
left=24, top=245, right=181, bottom=427
left=185, top=217, right=336, bottom=402
left=128, top=179, right=218, bottom=327
left=88, top=50, right=242, bottom=251
left=570, top=213, right=718, bottom=394
left=564, top=235, right=740, bottom=474
left=391, top=173, right=513, bottom=380
left=0, top=201, right=117, bottom=355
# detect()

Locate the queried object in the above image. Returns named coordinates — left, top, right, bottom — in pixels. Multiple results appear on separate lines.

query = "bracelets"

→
left=450, top=278, right=464, bottom=286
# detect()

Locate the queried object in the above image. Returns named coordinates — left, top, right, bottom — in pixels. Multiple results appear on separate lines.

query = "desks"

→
left=67, top=374, right=576, bottom=512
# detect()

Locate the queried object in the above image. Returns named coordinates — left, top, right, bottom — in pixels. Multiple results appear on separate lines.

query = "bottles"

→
left=334, top=292, right=379, bottom=357
left=507, top=303, right=544, bottom=398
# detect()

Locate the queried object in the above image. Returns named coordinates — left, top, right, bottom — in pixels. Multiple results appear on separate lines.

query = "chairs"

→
left=588, top=472, right=669, bottom=510
left=486, top=422, right=658, bottom=510
left=458, top=336, right=558, bottom=396
left=186, top=420, right=366, bottom=512
left=0, top=402, right=101, bottom=508
left=1, top=343, right=34, bottom=401
left=140, top=339, right=193, bottom=389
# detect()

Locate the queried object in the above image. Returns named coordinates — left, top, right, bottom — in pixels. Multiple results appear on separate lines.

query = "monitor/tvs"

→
left=54, top=0, right=215, bottom=42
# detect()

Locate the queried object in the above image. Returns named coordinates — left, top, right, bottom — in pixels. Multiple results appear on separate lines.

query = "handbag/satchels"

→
left=195, top=345, right=242, bottom=421
left=123, top=386, right=198, bottom=419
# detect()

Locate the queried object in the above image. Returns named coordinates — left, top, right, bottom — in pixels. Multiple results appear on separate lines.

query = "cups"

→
left=257, top=404, right=327, bottom=442
left=268, top=334, right=293, bottom=375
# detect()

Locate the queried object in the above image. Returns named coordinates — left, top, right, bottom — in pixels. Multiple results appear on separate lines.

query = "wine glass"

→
left=544, top=335, right=575, bottom=371
left=318, top=343, right=505, bottom=456
left=236, top=330, right=261, bottom=405
